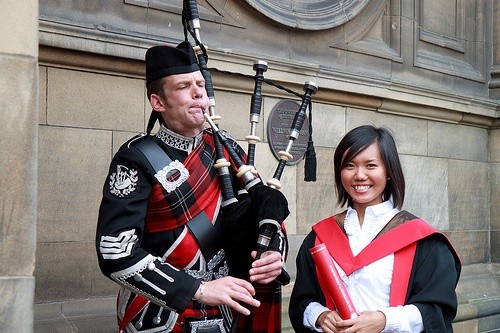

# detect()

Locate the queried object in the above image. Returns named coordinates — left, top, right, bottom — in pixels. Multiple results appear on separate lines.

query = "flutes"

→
left=180, top=0, right=317, bottom=333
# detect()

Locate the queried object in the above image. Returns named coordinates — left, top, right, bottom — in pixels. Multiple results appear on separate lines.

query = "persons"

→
left=96, top=41, right=291, bottom=333
left=288, top=125, right=461, bottom=333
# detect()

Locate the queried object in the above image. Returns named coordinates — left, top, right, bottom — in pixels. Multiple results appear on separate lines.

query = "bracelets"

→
left=197, top=281, right=206, bottom=304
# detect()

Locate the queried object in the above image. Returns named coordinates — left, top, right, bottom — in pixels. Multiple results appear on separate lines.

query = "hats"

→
left=145, top=41, right=199, bottom=86
